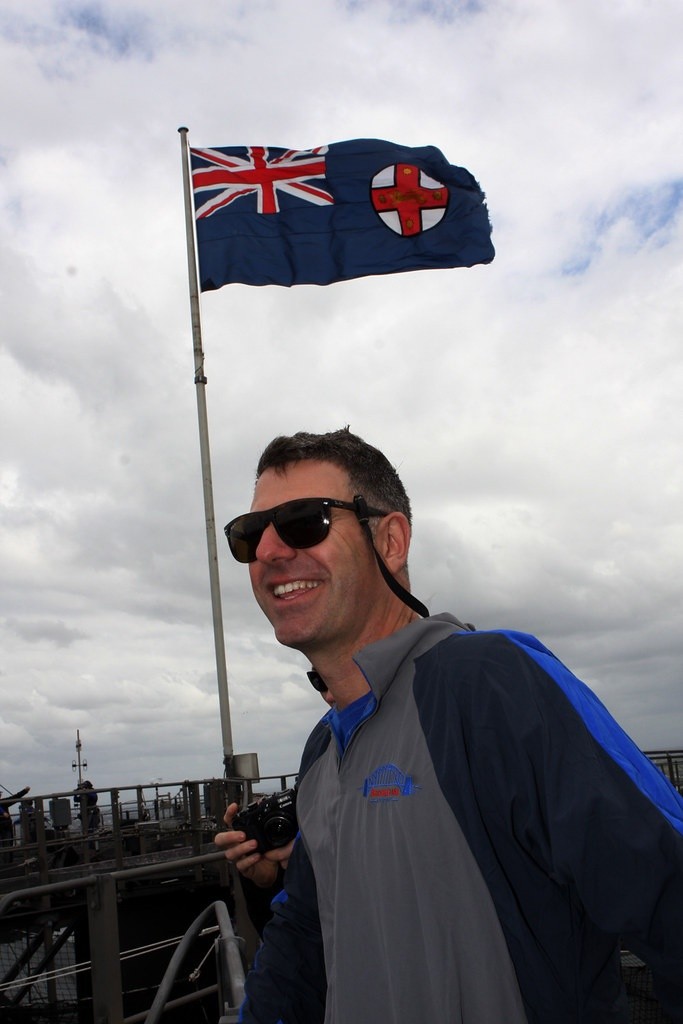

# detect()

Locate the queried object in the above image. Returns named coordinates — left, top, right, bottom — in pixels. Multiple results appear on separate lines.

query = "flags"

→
left=190, top=138, right=495, bottom=292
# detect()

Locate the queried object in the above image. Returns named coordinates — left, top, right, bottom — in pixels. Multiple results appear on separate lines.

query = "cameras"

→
left=232, top=789, right=302, bottom=855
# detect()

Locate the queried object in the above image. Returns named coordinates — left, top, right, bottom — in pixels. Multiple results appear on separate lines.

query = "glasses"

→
left=224, top=496, right=388, bottom=562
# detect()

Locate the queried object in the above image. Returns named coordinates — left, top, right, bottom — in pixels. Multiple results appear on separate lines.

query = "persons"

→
left=236, top=429, right=683, bottom=1024
left=73, top=781, right=98, bottom=848
left=214, top=666, right=336, bottom=941
left=12, top=801, right=35, bottom=843
left=0, top=787, right=30, bottom=862
